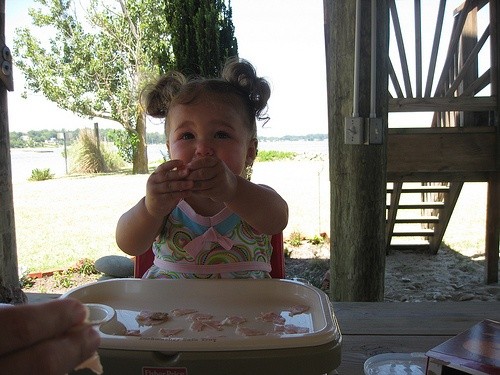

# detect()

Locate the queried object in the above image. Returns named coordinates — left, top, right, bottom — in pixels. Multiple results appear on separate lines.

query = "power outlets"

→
left=364, top=117, right=384, bottom=146
left=343, top=117, right=362, bottom=145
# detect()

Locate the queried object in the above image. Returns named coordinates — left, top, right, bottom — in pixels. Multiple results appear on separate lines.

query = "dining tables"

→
left=2, top=293, right=500, bottom=375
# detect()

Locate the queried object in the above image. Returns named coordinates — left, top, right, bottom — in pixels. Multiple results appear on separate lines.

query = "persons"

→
left=116, top=56, right=289, bottom=280
left=0, top=296, right=101, bottom=375
